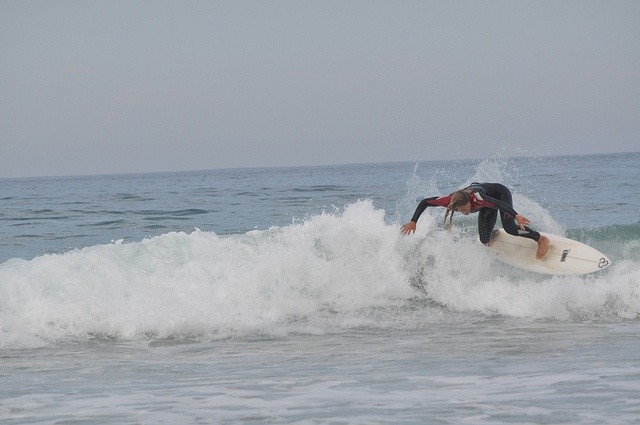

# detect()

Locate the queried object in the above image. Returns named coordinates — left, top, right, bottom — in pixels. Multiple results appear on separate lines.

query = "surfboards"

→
left=476, top=228, right=612, bottom=276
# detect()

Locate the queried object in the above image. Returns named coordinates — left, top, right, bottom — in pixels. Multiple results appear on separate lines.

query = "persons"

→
left=401, top=182, right=550, bottom=258
